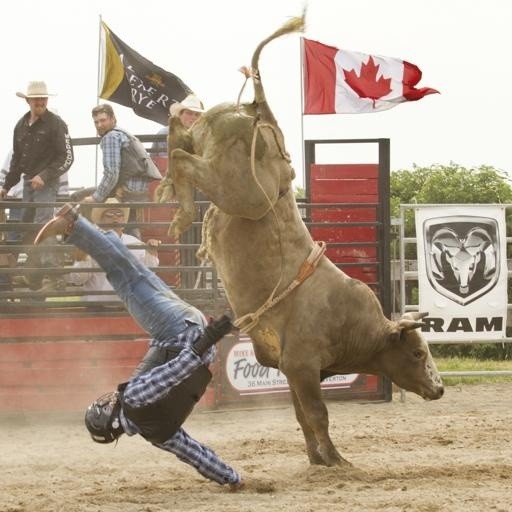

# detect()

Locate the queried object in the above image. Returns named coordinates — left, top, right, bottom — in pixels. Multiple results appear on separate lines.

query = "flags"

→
left=301, top=37, right=440, bottom=116
left=97, top=21, right=204, bottom=126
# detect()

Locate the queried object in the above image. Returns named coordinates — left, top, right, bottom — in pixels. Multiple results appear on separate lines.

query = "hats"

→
left=169, top=95, right=206, bottom=120
left=16, top=79, right=59, bottom=99
left=91, top=198, right=131, bottom=225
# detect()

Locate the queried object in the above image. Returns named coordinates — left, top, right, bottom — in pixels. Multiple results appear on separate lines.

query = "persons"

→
left=150, top=96, right=205, bottom=155
left=32, top=204, right=246, bottom=493
left=0, top=80, right=162, bottom=309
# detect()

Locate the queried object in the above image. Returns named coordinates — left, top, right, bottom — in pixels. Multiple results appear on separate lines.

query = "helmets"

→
left=84, top=391, right=124, bottom=443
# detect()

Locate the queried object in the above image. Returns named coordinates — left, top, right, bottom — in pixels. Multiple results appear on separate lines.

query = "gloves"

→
left=191, top=314, right=232, bottom=354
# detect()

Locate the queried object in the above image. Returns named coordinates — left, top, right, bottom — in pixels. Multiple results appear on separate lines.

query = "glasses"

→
left=105, top=212, right=123, bottom=217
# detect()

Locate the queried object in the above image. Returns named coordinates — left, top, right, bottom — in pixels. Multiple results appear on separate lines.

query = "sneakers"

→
left=34, top=204, right=78, bottom=246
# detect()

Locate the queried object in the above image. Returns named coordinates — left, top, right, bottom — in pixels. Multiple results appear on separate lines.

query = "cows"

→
left=152, top=7, right=445, bottom=467
